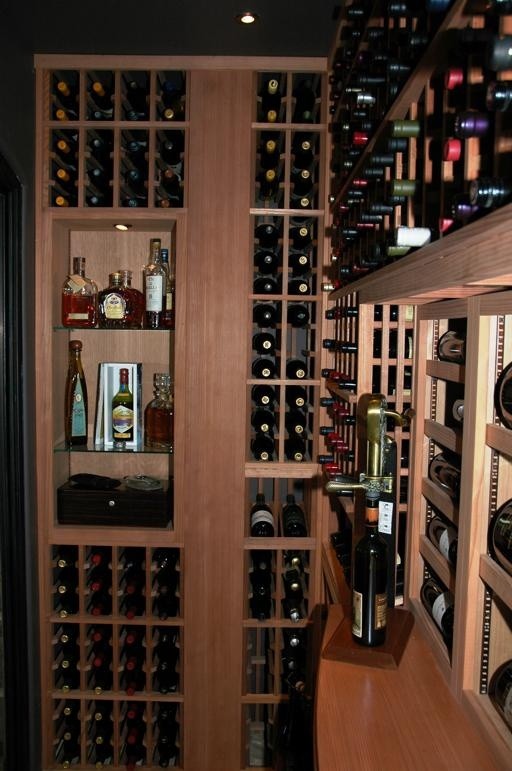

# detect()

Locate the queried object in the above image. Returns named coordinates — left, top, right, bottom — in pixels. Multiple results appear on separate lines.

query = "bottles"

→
left=63, top=339, right=89, bottom=446
left=251, top=76, right=314, bottom=462
left=250, top=490, right=308, bottom=695
left=321, top=0, right=511, bottom=730
left=61, top=237, right=173, bottom=330
left=50, top=71, right=184, bottom=207
left=144, top=373, right=173, bottom=448
left=56, top=553, right=177, bottom=769
left=112, top=366, right=134, bottom=444
